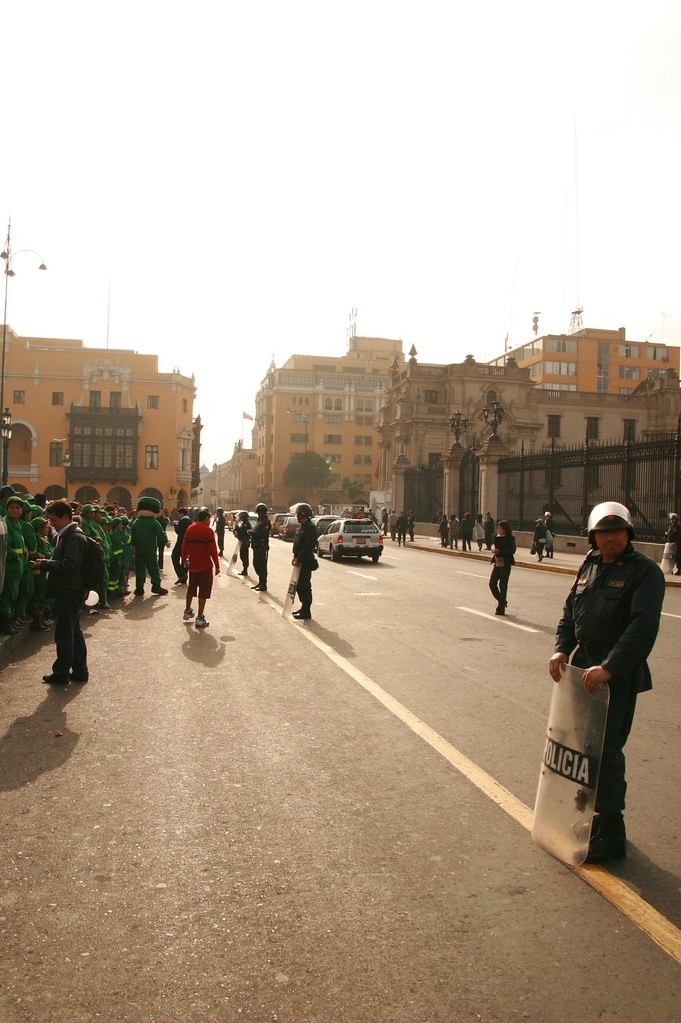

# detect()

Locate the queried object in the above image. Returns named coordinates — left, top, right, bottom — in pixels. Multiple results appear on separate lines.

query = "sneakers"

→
left=183, top=608, right=195, bottom=620
left=195, top=616, right=209, bottom=627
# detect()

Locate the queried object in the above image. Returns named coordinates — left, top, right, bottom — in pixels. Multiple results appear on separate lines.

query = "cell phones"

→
left=30, top=561, right=37, bottom=565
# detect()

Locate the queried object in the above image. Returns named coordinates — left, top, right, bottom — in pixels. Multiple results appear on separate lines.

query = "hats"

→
left=0, top=484, right=130, bottom=526
left=535, top=518, right=543, bottom=524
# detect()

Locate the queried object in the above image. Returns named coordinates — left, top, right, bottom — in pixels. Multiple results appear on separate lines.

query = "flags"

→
left=243, top=412, right=253, bottom=420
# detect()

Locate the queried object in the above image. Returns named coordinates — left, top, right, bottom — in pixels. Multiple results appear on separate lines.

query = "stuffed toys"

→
left=131, top=496, right=171, bottom=596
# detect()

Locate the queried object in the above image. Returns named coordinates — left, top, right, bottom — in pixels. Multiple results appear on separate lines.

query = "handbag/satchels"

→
left=530, top=541, right=536, bottom=555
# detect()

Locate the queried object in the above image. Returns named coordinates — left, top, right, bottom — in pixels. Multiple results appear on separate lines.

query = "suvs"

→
left=316, top=517, right=385, bottom=563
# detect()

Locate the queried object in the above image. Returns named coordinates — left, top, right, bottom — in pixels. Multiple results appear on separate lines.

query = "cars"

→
left=223, top=509, right=259, bottom=543
left=278, top=516, right=300, bottom=542
left=311, top=514, right=342, bottom=535
left=268, top=513, right=295, bottom=538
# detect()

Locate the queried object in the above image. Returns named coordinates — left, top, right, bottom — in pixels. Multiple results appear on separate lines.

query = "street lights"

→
left=1, top=407, right=13, bottom=486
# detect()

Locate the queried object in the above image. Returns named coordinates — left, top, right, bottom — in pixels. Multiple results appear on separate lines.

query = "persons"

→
left=0, top=485, right=57, bottom=634
left=33, top=500, right=95, bottom=684
left=184, top=506, right=209, bottom=523
left=180, top=511, right=220, bottom=626
left=548, top=501, right=667, bottom=861
left=533, top=510, right=556, bottom=562
left=488, top=520, right=517, bottom=615
left=381, top=508, right=415, bottom=547
left=61, top=498, right=138, bottom=614
left=232, top=511, right=251, bottom=576
left=289, top=501, right=319, bottom=619
left=340, top=507, right=379, bottom=526
left=247, top=502, right=272, bottom=590
left=666, top=513, right=681, bottom=575
left=435, top=511, right=494, bottom=552
left=214, top=507, right=225, bottom=556
left=171, top=506, right=192, bottom=585
left=155, top=509, right=170, bottom=571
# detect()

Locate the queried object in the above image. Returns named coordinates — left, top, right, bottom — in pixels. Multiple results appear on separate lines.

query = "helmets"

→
left=586, top=501, right=636, bottom=544
left=238, top=511, right=249, bottom=521
left=255, top=503, right=268, bottom=513
left=670, top=513, right=679, bottom=521
left=296, top=503, right=312, bottom=516
left=544, top=511, right=551, bottom=517
left=187, top=506, right=223, bottom=514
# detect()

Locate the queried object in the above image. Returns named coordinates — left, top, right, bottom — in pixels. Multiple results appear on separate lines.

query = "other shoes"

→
left=175, top=575, right=188, bottom=584
left=538, top=555, right=544, bottom=562
left=292, top=607, right=311, bottom=619
left=218, top=552, right=223, bottom=556
left=495, top=601, right=508, bottom=615
left=238, top=571, right=247, bottom=576
left=674, top=569, right=681, bottom=575
left=573, top=829, right=627, bottom=864
left=42, top=672, right=71, bottom=684
left=251, top=583, right=267, bottom=592
left=0, top=589, right=131, bottom=634
left=576, top=818, right=594, bottom=838
left=69, top=672, right=89, bottom=682
